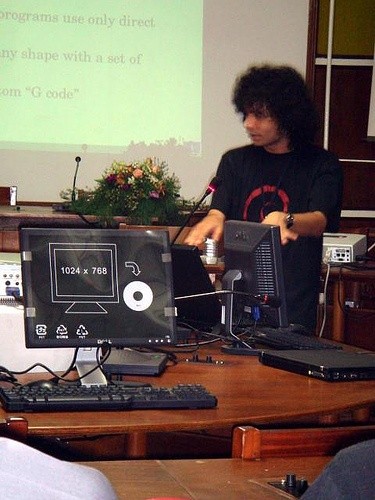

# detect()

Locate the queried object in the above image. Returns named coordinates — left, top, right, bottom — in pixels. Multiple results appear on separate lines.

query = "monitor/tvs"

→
left=18, top=224, right=176, bottom=387
left=221, top=219, right=290, bottom=356
left=169, top=243, right=222, bottom=339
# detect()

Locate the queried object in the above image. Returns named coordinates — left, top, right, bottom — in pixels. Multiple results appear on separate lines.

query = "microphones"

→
left=355, top=255, right=375, bottom=261
left=174, top=290, right=283, bottom=309
left=72, top=156, right=81, bottom=201
left=170, top=176, right=224, bottom=247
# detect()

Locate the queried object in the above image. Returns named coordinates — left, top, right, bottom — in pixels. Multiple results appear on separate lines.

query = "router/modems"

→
left=102, top=348, right=168, bottom=377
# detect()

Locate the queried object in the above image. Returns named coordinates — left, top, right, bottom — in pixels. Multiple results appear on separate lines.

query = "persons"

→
left=183, top=65, right=344, bottom=337
left=23, top=240, right=169, bottom=337
left=298, top=440, right=375, bottom=500
left=0, top=437, right=121, bottom=500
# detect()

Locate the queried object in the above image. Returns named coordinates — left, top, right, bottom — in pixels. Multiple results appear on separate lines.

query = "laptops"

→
left=259, top=347, right=374, bottom=382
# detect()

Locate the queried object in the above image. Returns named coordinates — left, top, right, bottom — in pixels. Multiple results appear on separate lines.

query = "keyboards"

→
left=254, top=331, right=344, bottom=351
left=0, top=383, right=217, bottom=412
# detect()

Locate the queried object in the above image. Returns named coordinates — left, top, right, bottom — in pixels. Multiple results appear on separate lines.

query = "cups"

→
left=10, top=187, right=17, bottom=206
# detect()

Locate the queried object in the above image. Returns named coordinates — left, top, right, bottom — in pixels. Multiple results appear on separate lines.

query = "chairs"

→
left=231, top=426, right=375, bottom=459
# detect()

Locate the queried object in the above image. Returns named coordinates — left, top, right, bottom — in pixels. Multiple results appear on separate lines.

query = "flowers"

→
left=55, top=157, right=211, bottom=226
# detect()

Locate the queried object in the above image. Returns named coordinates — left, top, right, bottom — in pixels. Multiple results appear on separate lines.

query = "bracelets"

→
left=286, top=212, right=293, bottom=229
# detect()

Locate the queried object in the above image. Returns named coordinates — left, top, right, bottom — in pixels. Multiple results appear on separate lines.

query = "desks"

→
left=0, top=332, right=375, bottom=458
left=319, top=260, right=375, bottom=342
left=0, top=201, right=207, bottom=252
left=66, top=457, right=336, bottom=500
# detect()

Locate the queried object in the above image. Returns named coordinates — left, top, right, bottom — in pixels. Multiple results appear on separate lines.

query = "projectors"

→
left=0, top=260, right=24, bottom=301
left=321, top=232, right=367, bottom=267
